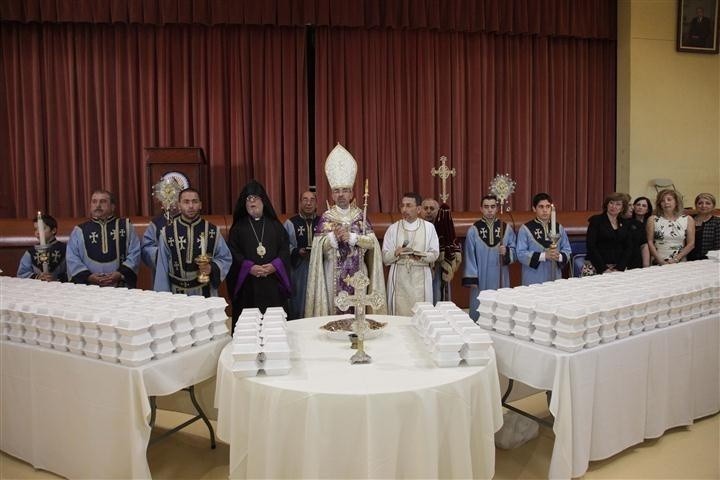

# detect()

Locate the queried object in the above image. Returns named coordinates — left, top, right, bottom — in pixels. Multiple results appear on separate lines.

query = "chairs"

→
left=652, top=178, right=685, bottom=209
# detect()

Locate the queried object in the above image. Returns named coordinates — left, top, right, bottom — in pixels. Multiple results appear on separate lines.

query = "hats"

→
left=324, top=141, right=358, bottom=190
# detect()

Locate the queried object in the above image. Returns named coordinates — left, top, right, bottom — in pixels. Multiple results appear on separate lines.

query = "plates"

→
left=320, top=318, right=388, bottom=340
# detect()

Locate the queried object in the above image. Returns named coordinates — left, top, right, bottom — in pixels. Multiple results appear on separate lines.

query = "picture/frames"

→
left=674, top=0, right=719, bottom=56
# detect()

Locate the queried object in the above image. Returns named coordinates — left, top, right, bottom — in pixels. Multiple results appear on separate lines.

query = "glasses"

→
left=246, top=195, right=263, bottom=201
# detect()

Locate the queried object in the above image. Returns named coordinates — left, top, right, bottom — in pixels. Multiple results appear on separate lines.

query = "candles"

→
left=550, top=204, right=559, bottom=234
left=198, top=235, right=206, bottom=256
left=35, top=218, right=46, bottom=244
